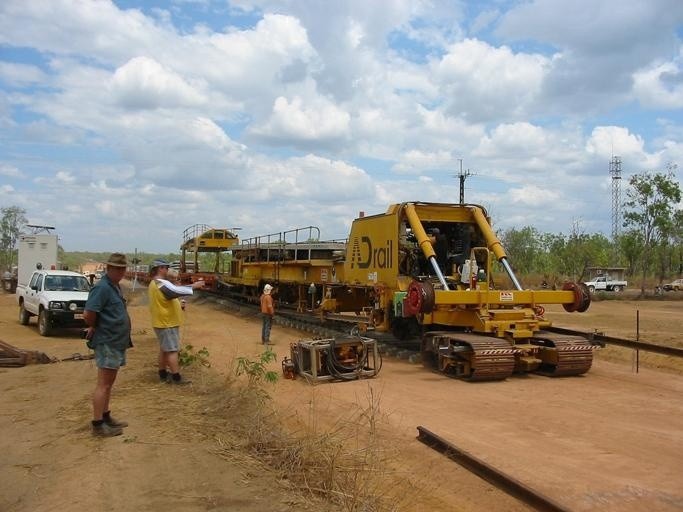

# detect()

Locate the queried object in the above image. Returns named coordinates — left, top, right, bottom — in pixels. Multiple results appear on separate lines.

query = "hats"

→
left=151, top=257, right=173, bottom=268
left=263, top=284, right=274, bottom=294
left=101, top=253, right=129, bottom=267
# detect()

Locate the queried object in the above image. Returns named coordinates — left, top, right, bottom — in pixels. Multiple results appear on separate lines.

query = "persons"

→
left=166, top=271, right=177, bottom=285
left=146, top=260, right=205, bottom=384
left=81, top=252, right=132, bottom=437
left=259, top=284, right=276, bottom=346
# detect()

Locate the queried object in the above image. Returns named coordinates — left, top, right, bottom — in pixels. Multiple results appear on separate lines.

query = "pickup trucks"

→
left=584, top=277, right=627, bottom=293
left=15, top=270, right=94, bottom=336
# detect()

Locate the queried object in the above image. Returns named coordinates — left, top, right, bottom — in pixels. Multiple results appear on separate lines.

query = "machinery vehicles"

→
left=121, top=201, right=593, bottom=383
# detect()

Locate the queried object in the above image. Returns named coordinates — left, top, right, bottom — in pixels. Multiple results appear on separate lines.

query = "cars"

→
left=2, top=268, right=18, bottom=293
left=663, top=279, right=683, bottom=292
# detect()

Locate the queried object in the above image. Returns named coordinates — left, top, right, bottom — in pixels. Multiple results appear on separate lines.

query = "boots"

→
left=92, top=420, right=122, bottom=436
left=172, top=372, right=192, bottom=384
left=159, top=369, right=172, bottom=383
left=104, top=410, right=128, bottom=428
